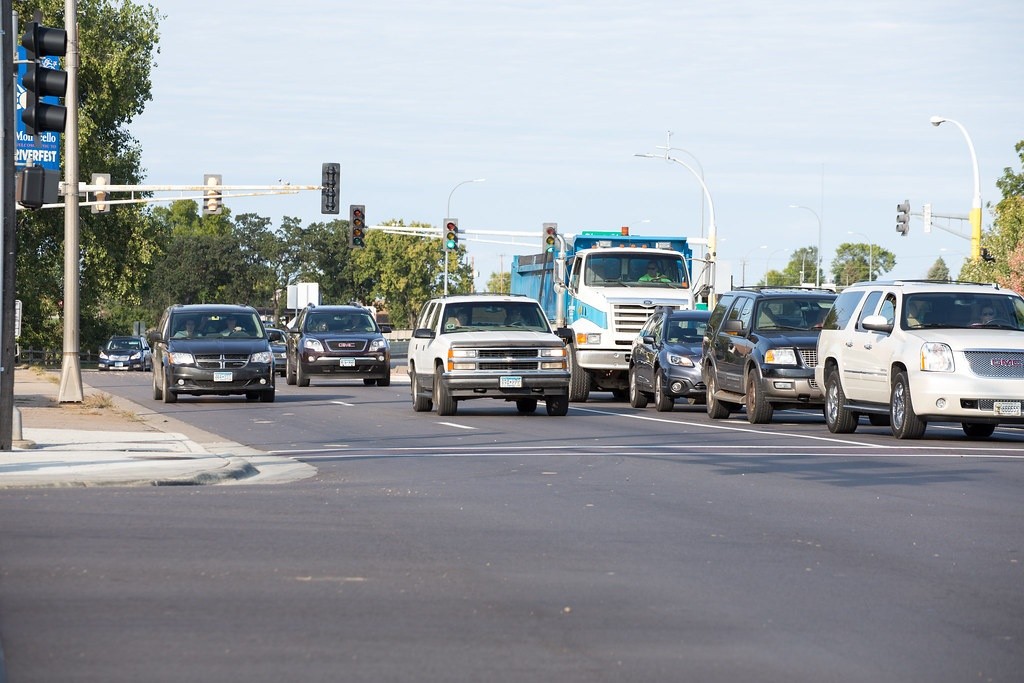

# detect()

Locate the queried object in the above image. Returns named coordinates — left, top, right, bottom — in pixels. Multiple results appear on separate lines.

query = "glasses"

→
left=509, top=311, right=521, bottom=317
left=983, top=311, right=993, bottom=316
left=646, top=265, right=657, bottom=270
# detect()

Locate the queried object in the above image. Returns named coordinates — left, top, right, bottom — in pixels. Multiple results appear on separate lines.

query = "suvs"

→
left=814, top=278, right=1024, bottom=440
left=285, top=301, right=392, bottom=388
left=407, top=293, right=574, bottom=417
left=149, top=303, right=282, bottom=403
left=700, top=285, right=840, bottom=425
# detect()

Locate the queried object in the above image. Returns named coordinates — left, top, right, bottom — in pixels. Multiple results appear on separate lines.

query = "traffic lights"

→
left=348, top=205, right=366, bottom=249
left=443, top=218, right=459, bottom=253
left=21, top=21, right=68, bottom=136
left=542, top=223, right=558, bottom=254
left=895, top=199, right=910, bottom=236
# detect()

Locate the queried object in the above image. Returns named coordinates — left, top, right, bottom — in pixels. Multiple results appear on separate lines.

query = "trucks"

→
left=509, top=234, right=716, bottom=403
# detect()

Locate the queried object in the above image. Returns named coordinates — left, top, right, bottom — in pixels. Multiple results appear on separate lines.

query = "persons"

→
left=311, top=319, right=329, bottom=331
left=220, top=314, right=247, bottom=336
left=505, top=307, right=521, bottom=325
left=339, top=314, right=370, bottom=331
left=967, top=305, right=1008, bottom=325
left=760, top=308, right=792, bottom=326
left=268, top=333, right=279, bottom=342
left=114, top=342, right=121, bottom=348
left=637, top=260, right=671, bottom=283
left=173, top=318, right=203, bottom=338
left=457, top=309, right=468, bottom=326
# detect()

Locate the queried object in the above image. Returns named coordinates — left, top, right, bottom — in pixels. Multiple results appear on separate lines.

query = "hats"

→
left=227, top=315, right=235, bottom=321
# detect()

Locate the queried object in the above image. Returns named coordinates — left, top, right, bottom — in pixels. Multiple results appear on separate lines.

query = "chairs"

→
left=925, top=303, right=997, bottom=327
left=587, top=265, right=606, bottom=281
left=907, top=304, right=922, bottom=328
left=446, top=310, right=469, bottom=326
left=671, top=326, right=698, bottom=341
left=807, top=309, right=829, bottom=326
left=176, top=319, right=187, bottom=332
left=759, top=308, right=781, bottom=328
left=314, top=321, right=329, bottom=331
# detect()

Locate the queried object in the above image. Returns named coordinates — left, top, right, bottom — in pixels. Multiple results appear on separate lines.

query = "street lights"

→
left=742, top=245, right=768, bottom=287
left=764, top=248, right=789, bottom=286
left=655, top=145, right=706, bottom=285
left=443, top=178, right=488, bottom=296
left=929, top=115, right=983, bottom=262
left=633, top=153, right=720, bottom=311
left=802, top=249, right=813, bottom=282
left=790, top=204, right=822, bottom=287
left=847, top=231, right=872, bottom=282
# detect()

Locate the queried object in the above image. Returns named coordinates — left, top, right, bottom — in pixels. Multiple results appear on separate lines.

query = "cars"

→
left=628, top=307, right=713, bottom=413
left=98, top=334, right=153, bottom=372
left=263, top=328, right=289, bottom=378
